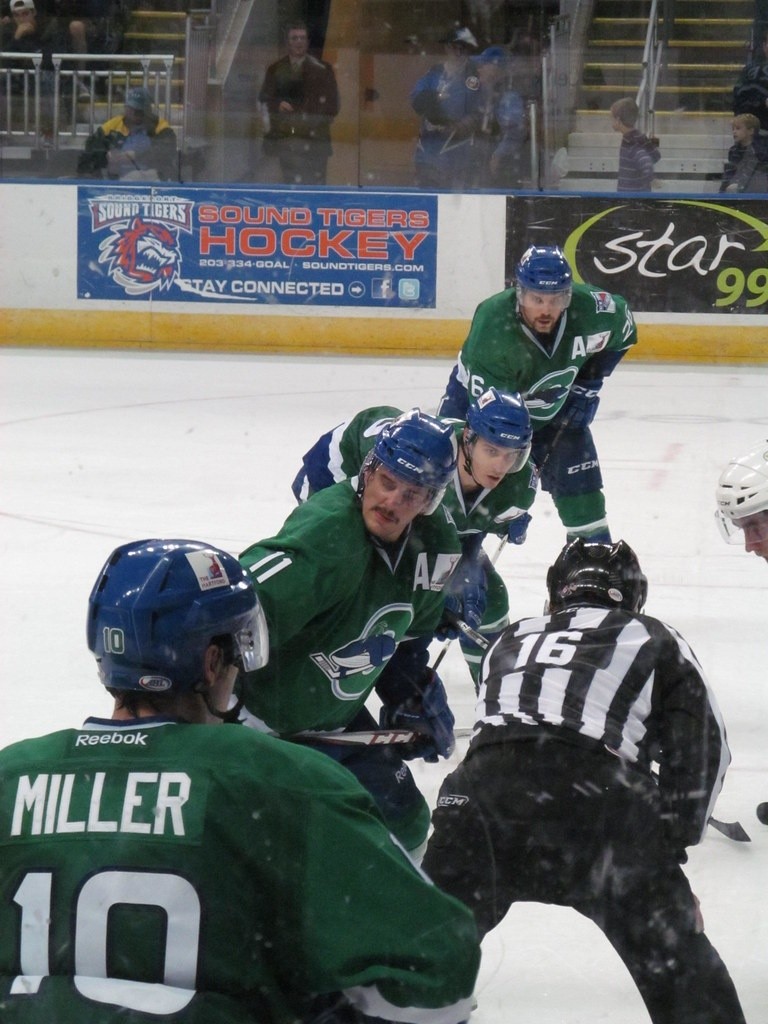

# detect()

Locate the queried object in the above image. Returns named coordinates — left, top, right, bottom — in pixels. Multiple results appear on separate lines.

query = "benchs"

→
left=573, top=0, right=754, bottom=119
left=0, top=0, right=209, bottom=145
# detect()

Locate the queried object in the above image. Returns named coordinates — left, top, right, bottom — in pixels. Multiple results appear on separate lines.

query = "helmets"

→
left=466, top=386, right=534, bottom=449
left=514, top=244, right=573, bottom=292
left=373, top=407, right=458, bottom=486
left=87, top=539, right=256, bottom=693
left=717, top=441, right=768, bottom=519
left=546, top=536, right=648, bottom=614
left=443, top=27, right=477, bottom=49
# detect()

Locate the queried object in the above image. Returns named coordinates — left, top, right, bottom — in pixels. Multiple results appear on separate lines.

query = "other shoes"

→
left=78, top=85, right=89, bottom=102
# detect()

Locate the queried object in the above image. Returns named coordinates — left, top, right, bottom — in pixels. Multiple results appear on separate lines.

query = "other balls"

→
left=756, top=802, right=768, bottom=826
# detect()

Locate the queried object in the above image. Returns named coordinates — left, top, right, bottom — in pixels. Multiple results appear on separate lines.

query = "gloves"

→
left=494, top=513, right=533, bottom=545
left=550, top=378, right=603, bottom=431
left=434, top=562, right=488, bottom=642
left=375, top=650, right=456, bottom=763
left=448, top=114, right=478, bottom=140
left=416, top=89, right=443, bottom=113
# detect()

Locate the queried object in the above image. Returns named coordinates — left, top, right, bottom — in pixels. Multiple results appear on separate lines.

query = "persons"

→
left=411, top=0, right=559, bottom=191
left=236, top=408, right=465, bottom=856
left=419, top=536, right=749, bottom=1024
left=77, top=89, right=181, bottom=183
left=258, top=24, right=339, bottom=185
left=435, top=243, right=636, bottom=546
left=713, top=442, right=768, bottom=824
left=1, top=0, right=127, bottom=102
left=609, top=96, right=664, bottom=192
left=718, top=31, right=768, bottom=193
left=1, top=537, right=481, bottom=1024
left=289, top=387, right=540, bottom=695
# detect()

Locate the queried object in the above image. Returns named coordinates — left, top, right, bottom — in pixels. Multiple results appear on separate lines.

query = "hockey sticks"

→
left=538, top=403, right=577, bottom=475
left=283, top=726, right=476, bottom=749
left=431, top=532, right=510, bottom=671
left=456, top=616, right=753, bottom=844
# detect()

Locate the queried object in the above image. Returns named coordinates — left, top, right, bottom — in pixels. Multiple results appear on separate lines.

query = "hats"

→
left=470, top=45, right=507, bottom=65
left=10, top=0, right=34, bottom=13
left=127, top=87, right=150, bottom=112
